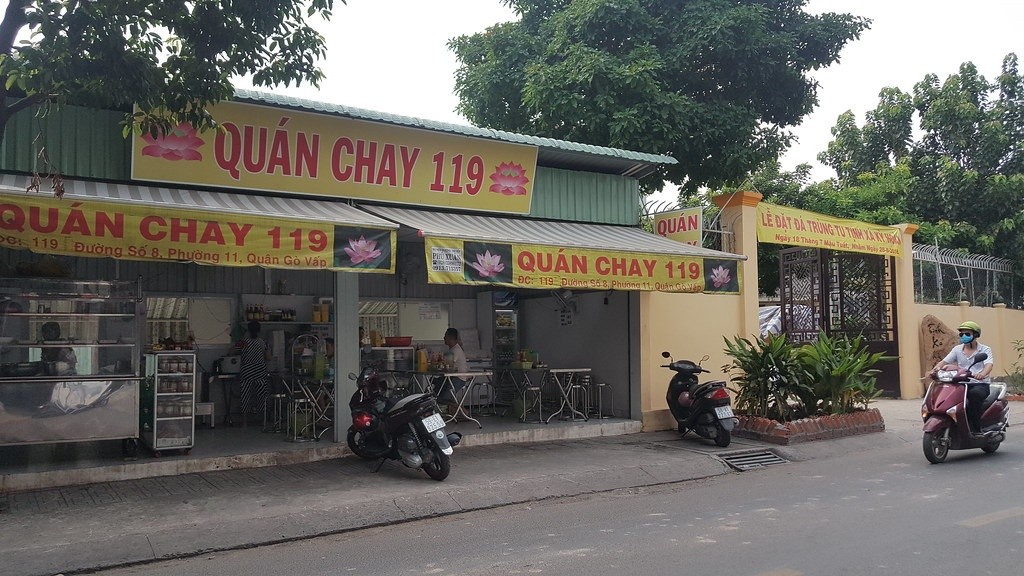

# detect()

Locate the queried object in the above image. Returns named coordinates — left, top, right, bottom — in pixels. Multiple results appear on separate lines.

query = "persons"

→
left=434, top=328, right=467, bottom=404
left=228, top=321, right=272, bottom=432
left=41, top=323, right=78, bottom=369
left=925, top=321, right=994, bottom=417
left=325, top=326, right=365, bottom=358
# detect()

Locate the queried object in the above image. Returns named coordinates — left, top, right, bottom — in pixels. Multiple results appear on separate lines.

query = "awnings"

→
left=0, top=172, right=401, bottom=274
left=356, top=204, right=747, bottom=295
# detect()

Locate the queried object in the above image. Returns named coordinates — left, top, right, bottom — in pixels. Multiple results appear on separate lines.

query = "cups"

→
left=444, top=351, right=454, bottom=366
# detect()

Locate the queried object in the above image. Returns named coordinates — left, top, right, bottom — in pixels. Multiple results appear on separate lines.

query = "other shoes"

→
left=973, top=429, right=984, bottom=438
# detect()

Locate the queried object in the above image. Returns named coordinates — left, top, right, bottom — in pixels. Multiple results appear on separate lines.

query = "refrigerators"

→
left=477, top=291, right=522, bottom=404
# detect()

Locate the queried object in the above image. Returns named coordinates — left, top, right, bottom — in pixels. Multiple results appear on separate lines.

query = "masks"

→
left=960, top=335, right=977, bottom=343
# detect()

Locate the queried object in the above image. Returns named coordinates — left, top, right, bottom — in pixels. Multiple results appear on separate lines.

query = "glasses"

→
left=959, top=332, right=974, bottom=337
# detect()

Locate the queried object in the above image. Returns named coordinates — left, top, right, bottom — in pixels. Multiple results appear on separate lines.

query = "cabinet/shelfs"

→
left=141, top=353, right=196, bottom=456
left=0, top=275, right=143, bottom=462
left=360, top=347, right=415, bottom=396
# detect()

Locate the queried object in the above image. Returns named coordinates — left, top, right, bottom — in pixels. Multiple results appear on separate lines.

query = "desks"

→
left=215, top=374, right=238, bottom=427
left=275, top=371, right=334, bottom=442
left=494, top=367, right=528, bottom=418
left=542, top=370, right=591, bottom=423
left=384, top=369, right=493, bottom=429
left=195, top=402, right=215, bottom=428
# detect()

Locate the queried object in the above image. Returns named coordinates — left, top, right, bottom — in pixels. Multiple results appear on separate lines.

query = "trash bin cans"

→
left=512, top=399, right=527, bottom=418
left=290, top=413, right=311, bottom=437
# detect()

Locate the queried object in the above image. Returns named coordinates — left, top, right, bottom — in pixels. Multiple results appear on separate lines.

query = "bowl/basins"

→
left=4, top=363, right=39, bottom=377
left=385, top=336, right=411, bottom=346
left=0, top=337, right=14, bottom=343
left=45, top=361, right=71, bottom=375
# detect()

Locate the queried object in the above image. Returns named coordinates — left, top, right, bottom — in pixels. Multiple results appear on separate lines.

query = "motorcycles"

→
left=345, top=343, right=463, bottom=483
left=916, top=353, right=1010, bottom=465
left=661, top=350, right=735, bottom=450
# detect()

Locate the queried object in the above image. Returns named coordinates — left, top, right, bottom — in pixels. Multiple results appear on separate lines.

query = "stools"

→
left=522, top=370, right=614, bottom=424
left=286, top=399, right=317, bottom=443
left=387, top=387, right=406, bottom=398
left=472, top=382, right=498, bottom=416
left=448, top=386, right=466, bottom=421
left=262, top=394, right=287, bottom=432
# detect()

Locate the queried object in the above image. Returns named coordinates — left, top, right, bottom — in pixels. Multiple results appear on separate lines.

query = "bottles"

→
left=416, top=343, right=426, bottom=351
left=246, top=304, right=264, bottom=321
left=280, top=308, right=296, bottom=322
left=157, top=360, right=193, bottom=417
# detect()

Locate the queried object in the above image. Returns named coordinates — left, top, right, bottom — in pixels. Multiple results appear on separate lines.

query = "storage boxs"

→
left=369, top=329, right=380, bottom=347
left=313, top=355, right=324, bottom=378
left=313, top=311, right=321, bottom=322
left=418, top=350, right=427, bottom=372
left=321, top=303, right=329, bottom=322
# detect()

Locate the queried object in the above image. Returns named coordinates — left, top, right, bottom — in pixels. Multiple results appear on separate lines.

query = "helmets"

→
left=678, top=391, right=692, bottom=407
left=957, top=321, right=981, bottom=339
left=352, top=411, right=377, bottom=432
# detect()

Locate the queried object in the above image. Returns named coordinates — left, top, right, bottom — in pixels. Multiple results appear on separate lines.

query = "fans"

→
left=550, top=289, right=580, bottom=312
left=395, top=253, right=422, bottom=285
